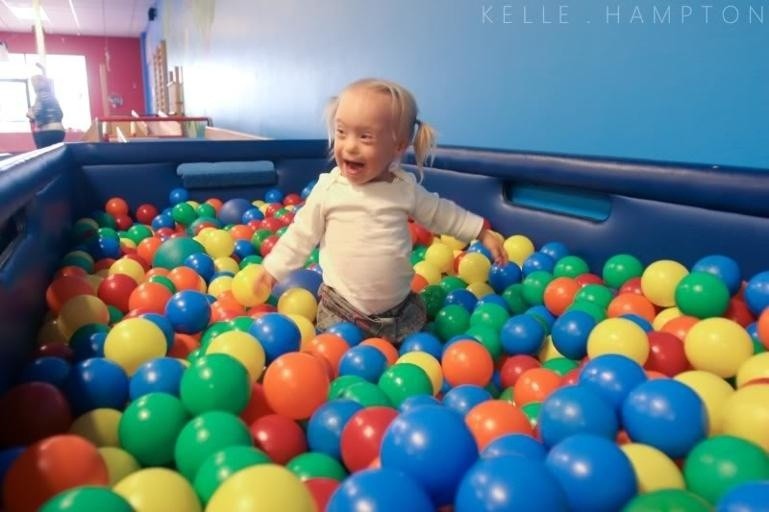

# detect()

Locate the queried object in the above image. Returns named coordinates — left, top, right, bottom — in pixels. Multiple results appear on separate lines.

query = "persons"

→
left=25, top=74, right=66, bottom=149
left=252, top=80, right=507, bottom=345
